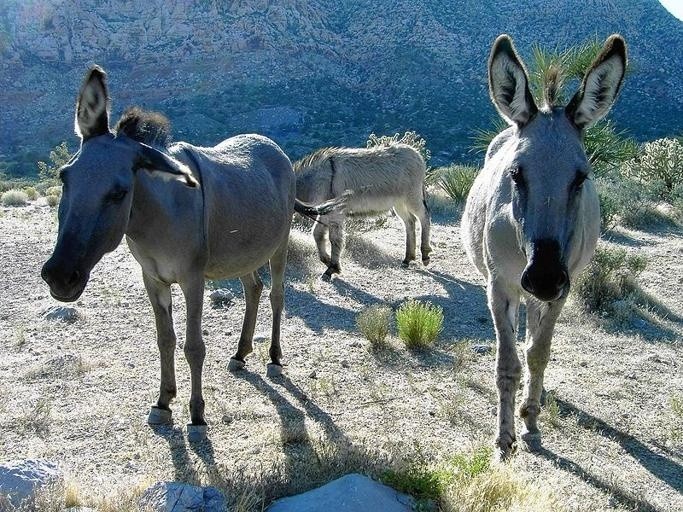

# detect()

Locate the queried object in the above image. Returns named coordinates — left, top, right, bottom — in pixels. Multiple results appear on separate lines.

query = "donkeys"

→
left=40, top=62, right=355, bottom=445
left=459, top=32, right=628, bottom=464
left=294, top=142, right=431, bottom=283
